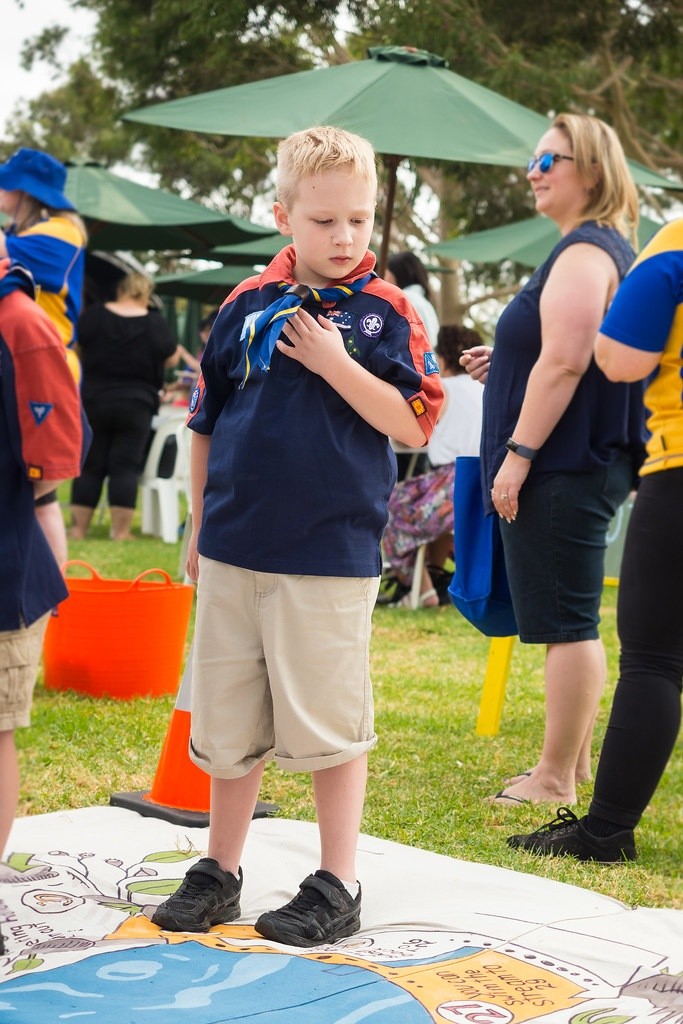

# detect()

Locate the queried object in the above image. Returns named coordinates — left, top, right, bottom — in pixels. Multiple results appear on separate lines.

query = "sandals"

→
left=387, top=590, right=437, bottom=608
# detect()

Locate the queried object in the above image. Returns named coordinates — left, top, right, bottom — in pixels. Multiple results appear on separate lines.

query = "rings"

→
left=501, top=494, right=507, bottom=497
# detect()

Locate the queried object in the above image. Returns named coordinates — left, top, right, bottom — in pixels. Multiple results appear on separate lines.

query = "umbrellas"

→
left=419, top=212, right=661, bottom=270
left=162, top=231, right=293, bottom=264
left=0, top=154, right=280, bottom=252
left=120, top=45, right=683, bottom=277
left=150, top=267, right=262, bottom=305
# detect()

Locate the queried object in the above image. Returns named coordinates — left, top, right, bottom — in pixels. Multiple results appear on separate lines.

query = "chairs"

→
left=143, top=406, right=193, bottom=544
left=475, top=579, right=621, bottom=740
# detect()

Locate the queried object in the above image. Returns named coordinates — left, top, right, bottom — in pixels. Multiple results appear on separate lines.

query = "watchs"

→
left=505, top=437, right=540, bottom=461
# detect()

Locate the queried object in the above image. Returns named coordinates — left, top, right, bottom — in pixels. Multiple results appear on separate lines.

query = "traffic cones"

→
left=108, top=625, right=284, bottom=829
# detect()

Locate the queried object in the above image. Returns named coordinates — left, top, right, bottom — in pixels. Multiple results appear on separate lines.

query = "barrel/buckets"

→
left=42, top=560, right=196, bottom=700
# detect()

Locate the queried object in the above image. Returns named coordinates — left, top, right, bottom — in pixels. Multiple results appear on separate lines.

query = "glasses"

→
left=529, top=153, right=572, bottom=172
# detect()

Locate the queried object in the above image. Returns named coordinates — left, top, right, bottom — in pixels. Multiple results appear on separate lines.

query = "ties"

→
left=231, top=269, right=380, bottom=391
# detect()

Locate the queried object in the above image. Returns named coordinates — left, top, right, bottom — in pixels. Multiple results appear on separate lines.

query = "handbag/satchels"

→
left=445, top=456, right=518, bottom=638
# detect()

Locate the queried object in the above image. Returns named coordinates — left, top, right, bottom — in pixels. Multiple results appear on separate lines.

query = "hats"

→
left=0, top=147, right=77, bottom=213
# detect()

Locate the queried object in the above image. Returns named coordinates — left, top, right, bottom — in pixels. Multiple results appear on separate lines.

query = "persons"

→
left=503, top=214, right=683, bottom=864
left=376, top=253, right=482, bottom=606
left=0, top=147, right=88, bottom=863
left=65, top=273, right=220, bottom=540
left=459, top=113, right=660, bottom=809
left=150, top=125, right=445, bottom=948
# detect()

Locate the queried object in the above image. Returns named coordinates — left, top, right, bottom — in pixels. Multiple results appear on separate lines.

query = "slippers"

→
left=503, top=773, right=529, bottom=785
left=495, top=792, right=525, bottom=805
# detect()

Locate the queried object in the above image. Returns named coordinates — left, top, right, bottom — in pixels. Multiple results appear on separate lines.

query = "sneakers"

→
left=508, top=807, right=637, bottom=863
left=152, top=858, right=245, bottom=932
left=254, top=870, right=362, bottom=947
